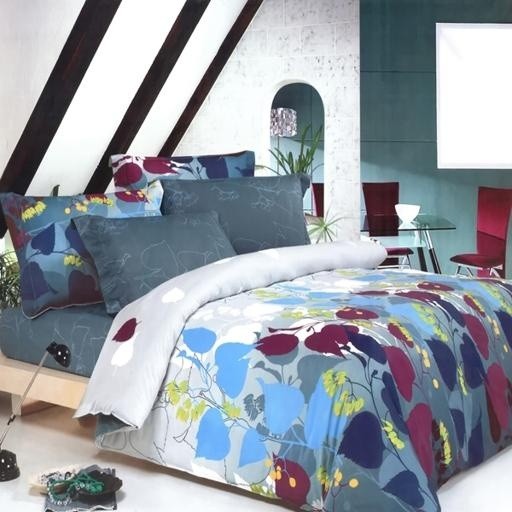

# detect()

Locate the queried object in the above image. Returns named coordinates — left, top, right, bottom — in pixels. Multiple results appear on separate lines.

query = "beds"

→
left=1, top=239, right=511, bottom=512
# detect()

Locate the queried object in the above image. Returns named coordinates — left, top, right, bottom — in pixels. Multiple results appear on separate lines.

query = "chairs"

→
left=449, top=186, right=512, bottom=278
left=312, top=182, right=323, bottom=218
left=363, top=180, right=414, bottom=269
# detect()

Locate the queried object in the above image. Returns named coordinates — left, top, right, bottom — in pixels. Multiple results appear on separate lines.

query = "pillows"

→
left=70, top=210, right=238, bottom=315
left=108, top=151, right=256, bottom=208
left=1, top=180, right=165, bottom=320
left=157, top=172, right=312, bottom=256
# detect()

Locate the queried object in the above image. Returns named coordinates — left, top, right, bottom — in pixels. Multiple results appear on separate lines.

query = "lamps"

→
left=1, top=340, right=72, bottom=481
left=271, top=107, right=298, bottom=175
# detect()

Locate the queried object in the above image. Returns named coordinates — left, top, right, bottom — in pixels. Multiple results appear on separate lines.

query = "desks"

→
left=361, top=212, right=455, bottom=274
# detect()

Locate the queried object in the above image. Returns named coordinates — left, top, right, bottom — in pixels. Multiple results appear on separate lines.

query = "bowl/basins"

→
left=395, top=204, right=420, bottom=222
